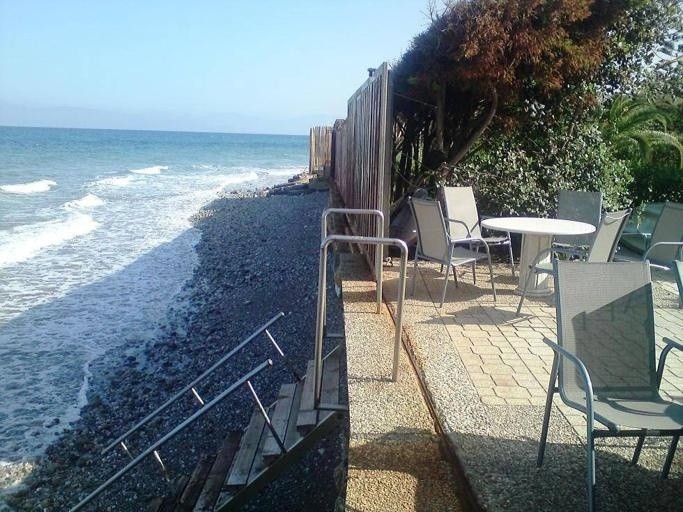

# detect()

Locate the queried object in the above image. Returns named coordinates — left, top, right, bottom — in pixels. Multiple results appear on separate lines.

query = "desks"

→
left=478, top=215, right=598, bottom=298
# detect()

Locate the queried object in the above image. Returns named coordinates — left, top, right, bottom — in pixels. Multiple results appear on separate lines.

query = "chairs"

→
left=612, top=199, right=681, bottom=315
left=536, top=258, right=681, bottom=511
left=549, top=190, right=604, bottom=265
left=437, top=186, right=516, bottom=290
left=407, top=195, right=499, bottom=310
left=513, top=207, right=635, bottom=330
